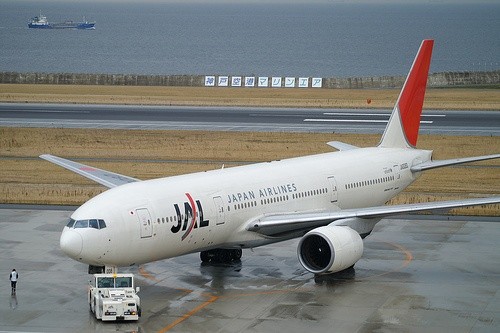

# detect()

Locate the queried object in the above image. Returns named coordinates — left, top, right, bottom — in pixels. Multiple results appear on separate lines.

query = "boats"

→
left=28, top=15, right=48, bottom=28
left=77, top=21, right=96, bottom=30
left=48, top=20, right=83, bottom=29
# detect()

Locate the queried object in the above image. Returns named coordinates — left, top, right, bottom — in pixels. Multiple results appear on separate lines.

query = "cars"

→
left=88, top=273, right=141, bottom=321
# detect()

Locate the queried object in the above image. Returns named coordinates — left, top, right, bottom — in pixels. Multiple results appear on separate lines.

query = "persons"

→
left=10, top=268, right=19, bottom=291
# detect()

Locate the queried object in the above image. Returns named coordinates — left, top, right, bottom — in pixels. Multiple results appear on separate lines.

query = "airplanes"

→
left=37, top=39, right=500, bottom=276
